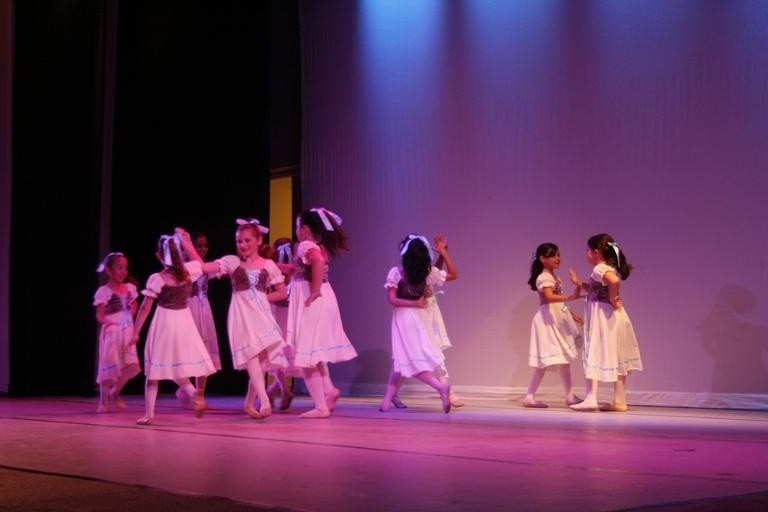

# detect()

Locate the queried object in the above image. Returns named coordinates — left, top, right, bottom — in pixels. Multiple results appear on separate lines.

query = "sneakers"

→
left=565, top=395, right=583, bottom=405
left=391, top=395, right=407, bottom=408
left=569, top=402, right=599, bottom=412
left=440, top=382, right=451, bottom=413
left=449, top=398, right=464, bottom=407
left=379, top=402, right=389, bottom=412
left=522, top=399, right=548, bottom=408
left=600, top=404, right=628, bottom=412
left=96, top=388, right=341, bottom=424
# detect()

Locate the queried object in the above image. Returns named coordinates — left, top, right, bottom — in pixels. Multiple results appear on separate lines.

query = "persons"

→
left=568, top=233, right=644, bottom=411
left=127, top=207, right=360, bottom=425
left=379, top=232, right=464, bottom=414
left=93, top=252, right=141, bottom=414
left=522, top=242, right=587, bottom=409
